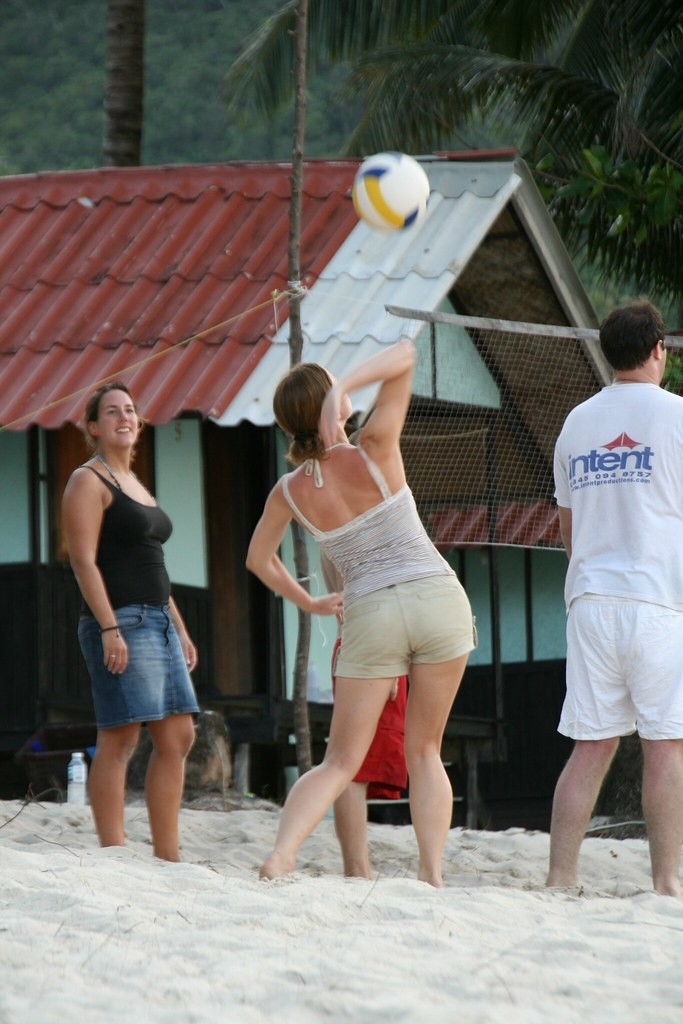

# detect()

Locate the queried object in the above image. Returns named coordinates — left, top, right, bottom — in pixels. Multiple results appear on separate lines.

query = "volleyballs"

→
left=348, top=153, right=432, bottom=231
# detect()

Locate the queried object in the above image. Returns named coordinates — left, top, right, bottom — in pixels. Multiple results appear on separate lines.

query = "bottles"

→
left=66, top=753, right=87, bottom=803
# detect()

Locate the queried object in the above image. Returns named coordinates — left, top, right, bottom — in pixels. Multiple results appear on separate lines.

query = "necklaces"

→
left=96, top=454, right=149, bottom=493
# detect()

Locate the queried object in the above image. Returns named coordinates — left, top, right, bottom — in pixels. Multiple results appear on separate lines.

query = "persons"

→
left=60, top=383, right=202, bottom=863
left=543, top=297, right=683, bottom=900
left=246, top=338, right=476, bottom=891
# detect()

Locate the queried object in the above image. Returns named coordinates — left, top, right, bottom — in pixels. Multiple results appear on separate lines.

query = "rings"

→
left=110, top=655, right=116, bottom=658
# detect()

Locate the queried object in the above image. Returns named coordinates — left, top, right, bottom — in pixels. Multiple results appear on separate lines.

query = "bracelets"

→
left=99, top=625, right=119, bottom=633
